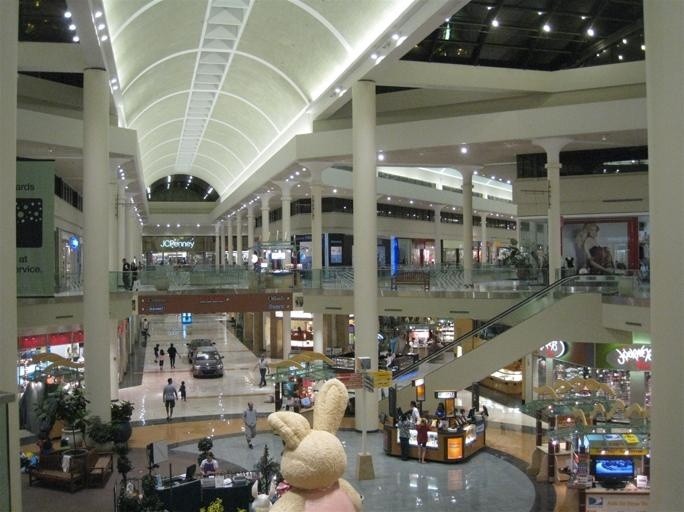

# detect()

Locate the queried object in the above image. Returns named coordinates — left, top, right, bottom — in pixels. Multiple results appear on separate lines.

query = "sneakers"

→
left=249, top=443, right=253, bottom=449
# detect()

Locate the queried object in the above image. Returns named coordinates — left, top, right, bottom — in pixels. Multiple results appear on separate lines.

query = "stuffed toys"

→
left=265, top=377, right=362, bottom=512
left=251, top=479, right=275, bottom=512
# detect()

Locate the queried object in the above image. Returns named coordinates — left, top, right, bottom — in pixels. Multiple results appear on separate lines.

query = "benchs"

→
left=391, top=269, right=430, bottom=291
left=87, top=452, right=113, bottom=488
left=28, top=464, right=84, bottom=494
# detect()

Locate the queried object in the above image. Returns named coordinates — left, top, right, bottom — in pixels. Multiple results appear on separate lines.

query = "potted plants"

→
left=501, top=240, right=533, bottom=279
left=33, top=386, right=134, bottom=453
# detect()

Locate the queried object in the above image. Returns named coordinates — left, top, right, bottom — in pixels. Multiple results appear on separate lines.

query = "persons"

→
left=384, top=353, right=392, bottom=368
left=253, top=353, right=270, bottom=388
left=154, top=344, right=160, bottom=363
left=199, top=451, right=219, bottom=476
left=159, top=349, right=165, bottom=370
left=584, top=222, right=615, bottom=275
left=25, top=455, right=38, bottom=472
left=167, top=343, right=181, bottom=369
left=143, top=318, right=150, bottom=337
left=121, top=258, right=131, bottom=290
left=162, top=377, right=178, bottom=420
left=242, top=401, right=257, bottom=449
left=130, top=262, right=138, bottom=290
left=385, top=348, right=392, bottom=358
left=20, top=448, right=28, bottom=469
left=178, top=381, right=187, bottom=401
left=397, top=400, right=445, bottom=464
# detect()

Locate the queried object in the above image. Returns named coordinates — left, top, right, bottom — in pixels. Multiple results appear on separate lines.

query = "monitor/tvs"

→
left=435, top=390, right=456, bottom=400
left=412, top=378, right=425, bottom=387
left=588, top=455, right=637, bottom=488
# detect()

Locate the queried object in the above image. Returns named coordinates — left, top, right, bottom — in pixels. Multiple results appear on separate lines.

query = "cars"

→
left=186, top=338, right=217, bottom=363
left=190, top=345, right=224, bottom=378
